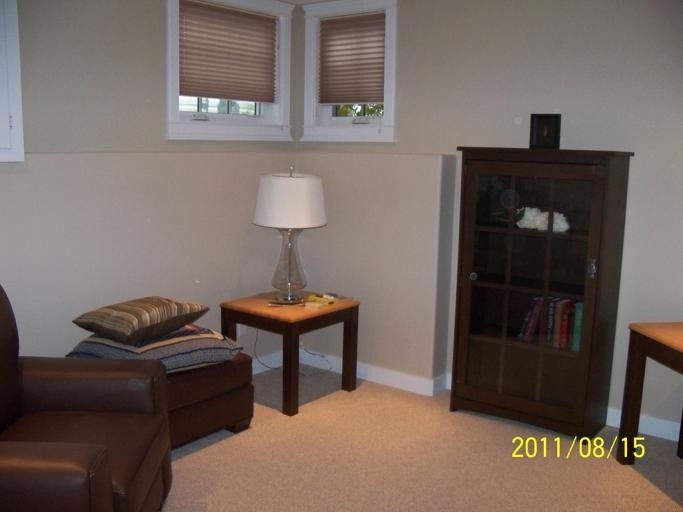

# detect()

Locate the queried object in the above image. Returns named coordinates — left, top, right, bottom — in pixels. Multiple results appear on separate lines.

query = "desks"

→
left=216, top=288, right=363, bottom=416
left=615, top=320, right=682, bottom=466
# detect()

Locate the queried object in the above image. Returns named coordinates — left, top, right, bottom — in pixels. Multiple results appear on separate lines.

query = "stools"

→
left=58, top=334, right=258, bottom=451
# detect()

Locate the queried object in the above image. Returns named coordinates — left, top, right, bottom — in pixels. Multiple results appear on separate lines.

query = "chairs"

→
left=0, top=285, right=174, bottom=511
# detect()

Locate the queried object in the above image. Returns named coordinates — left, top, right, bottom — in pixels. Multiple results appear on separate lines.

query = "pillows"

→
left=65, top=322, right=247, bottom=377
left=72, top=296, right=212, bottom=346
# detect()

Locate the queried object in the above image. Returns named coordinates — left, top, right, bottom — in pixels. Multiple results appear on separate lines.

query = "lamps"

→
left=253, top=162, right=328, bottom=306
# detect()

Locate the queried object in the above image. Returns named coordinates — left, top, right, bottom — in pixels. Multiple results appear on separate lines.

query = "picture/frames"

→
left=528, top=112, right=562, bottom=150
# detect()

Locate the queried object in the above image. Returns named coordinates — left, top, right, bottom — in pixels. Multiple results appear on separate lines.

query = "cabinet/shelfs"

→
left=450, top=146, right=635, bottom=443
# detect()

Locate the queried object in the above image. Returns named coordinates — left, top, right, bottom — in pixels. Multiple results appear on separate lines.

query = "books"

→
left=518, top=296, right=588, bottom=353
